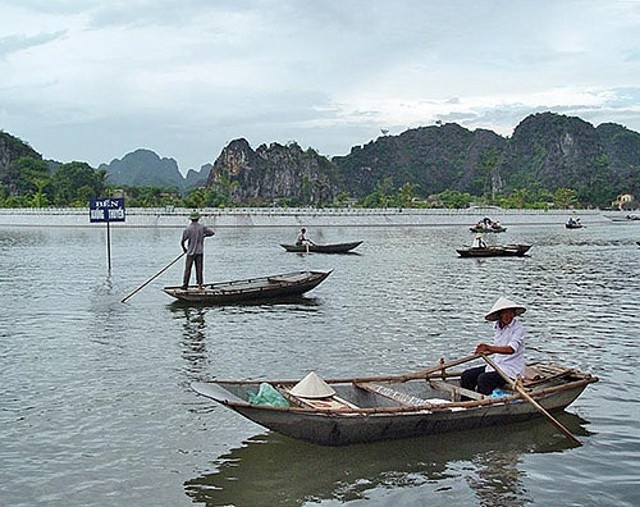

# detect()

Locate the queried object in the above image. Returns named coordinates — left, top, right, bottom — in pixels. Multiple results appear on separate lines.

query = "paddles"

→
left=480, top=352, right=581, bottom=445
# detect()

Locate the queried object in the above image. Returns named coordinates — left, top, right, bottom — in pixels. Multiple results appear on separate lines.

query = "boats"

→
left=280, top=241, right=363, bottom=254
left=190, top=365, right=599, bottom=446
left=162, top=269, right=335, bottom=301
left=566, top=223, right=581, bottom=228
left=469, top=226, right=507, bottom=232
left=455, top=244, right=531, bottom=255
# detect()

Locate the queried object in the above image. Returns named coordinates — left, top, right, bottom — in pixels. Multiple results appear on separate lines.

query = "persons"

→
left=181, top=210, right=215, bottom=291
left=493, top=220, right=501, bottom=228
left=485, top=219, right=493, bottom=229
left=461, top=296, right=526, bottom=401
left=296, top=228, right=310, bottom=245
left=568, top=217, right=576, bottom=225
left=475, top=219, right=485, bottom=229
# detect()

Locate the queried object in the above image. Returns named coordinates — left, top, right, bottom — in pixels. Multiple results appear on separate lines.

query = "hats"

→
left=485, top=296, right=526, bottom=320
left=188, top=211, right=200, bottom=219
left=289, top=370, right=336, bottom=398
